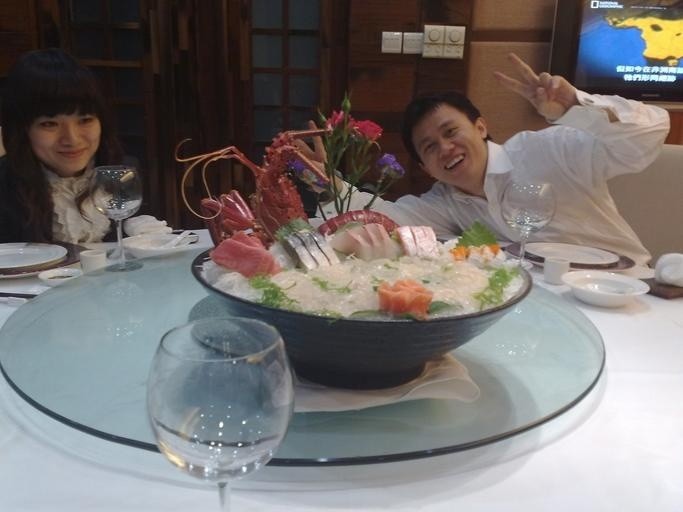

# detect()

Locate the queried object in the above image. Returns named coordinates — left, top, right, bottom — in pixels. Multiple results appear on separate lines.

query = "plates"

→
left=505, top=240, right=637, bottom=274
left=0, top=239, right=91, bottom=279
left=37, top=268, right=83, bottom=287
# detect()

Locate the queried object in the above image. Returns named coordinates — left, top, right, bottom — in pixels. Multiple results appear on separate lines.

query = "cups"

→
left=542, top=256, right=570, bottom=286
left=78, top=248, right=107, bottom=276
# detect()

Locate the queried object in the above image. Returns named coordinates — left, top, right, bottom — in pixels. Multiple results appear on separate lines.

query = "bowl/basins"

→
left=121, top=231, right=199, bottom=260
left=190, top=233, right=533, bottom=390
left=564, top=271, right=650, bottom=308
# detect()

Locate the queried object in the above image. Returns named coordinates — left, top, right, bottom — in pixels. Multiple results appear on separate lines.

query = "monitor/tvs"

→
left=541, top=0, right=683, bottom=119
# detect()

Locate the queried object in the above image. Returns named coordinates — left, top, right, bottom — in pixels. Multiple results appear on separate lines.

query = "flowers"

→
left=281, top=96, right=407, bottom=229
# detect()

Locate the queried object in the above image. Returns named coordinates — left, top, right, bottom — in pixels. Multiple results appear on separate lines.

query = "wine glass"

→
left=499, top=181, right=557, bottom=271
left=89, top=164, right=143, bottom=273
left=149, top=316, right=296, bottom=511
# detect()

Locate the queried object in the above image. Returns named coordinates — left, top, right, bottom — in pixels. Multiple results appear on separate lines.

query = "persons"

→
left=290, top=50, right=674, bottom=271
left=0, top=46, right=153, bottom=243
